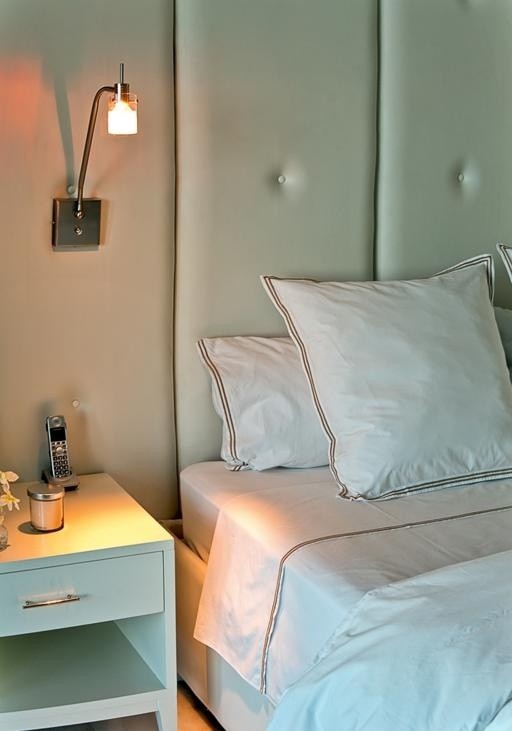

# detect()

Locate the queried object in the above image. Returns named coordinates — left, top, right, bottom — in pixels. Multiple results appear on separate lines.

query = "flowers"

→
left=0, top=470, right=20, bottom=524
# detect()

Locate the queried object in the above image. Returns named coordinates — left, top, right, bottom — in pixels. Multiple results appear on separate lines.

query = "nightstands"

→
left=0, top=474, right=178, bottom=731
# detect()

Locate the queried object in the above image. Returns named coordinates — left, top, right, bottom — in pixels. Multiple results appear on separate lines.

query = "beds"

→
left=160, top=460, right=512, bottom=731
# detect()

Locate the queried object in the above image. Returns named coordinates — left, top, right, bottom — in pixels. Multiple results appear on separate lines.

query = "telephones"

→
left=41, top=415, right=80, bottom=491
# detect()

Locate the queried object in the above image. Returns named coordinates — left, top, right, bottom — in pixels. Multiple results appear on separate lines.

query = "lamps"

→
left=53, top=64, right=137, bottom=250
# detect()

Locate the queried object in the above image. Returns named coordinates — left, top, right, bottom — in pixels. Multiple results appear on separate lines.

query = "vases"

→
left=0, top=525, right=8, bottom=551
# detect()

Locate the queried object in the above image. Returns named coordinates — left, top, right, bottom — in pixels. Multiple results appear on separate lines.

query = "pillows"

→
left=195, top=306, right=512, bottom=472
left=259, top=253, right=512, bottom=502
left=495, top=242, right=512, bottom=283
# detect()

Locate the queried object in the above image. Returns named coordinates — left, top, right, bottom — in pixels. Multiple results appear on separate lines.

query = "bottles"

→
left=27, top=482, right=65, bottom=532
